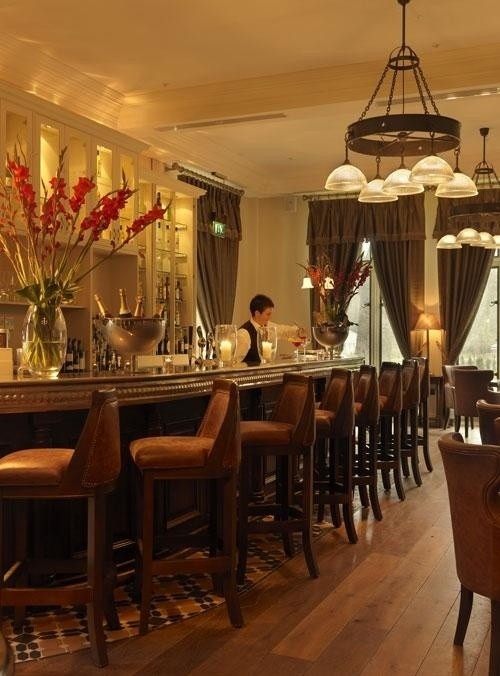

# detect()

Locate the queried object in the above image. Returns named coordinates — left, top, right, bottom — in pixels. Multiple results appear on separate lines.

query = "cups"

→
left=214, top=324, right=237, bottom=367
left=257, top=327, right=277, bottom=364
left=0, top=347, right=14, bottom=383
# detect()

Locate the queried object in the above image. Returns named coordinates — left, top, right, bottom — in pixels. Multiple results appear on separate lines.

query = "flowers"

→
left=296, top=250, right=379, bottom=324
left=0, top=137, right=171, bottom=368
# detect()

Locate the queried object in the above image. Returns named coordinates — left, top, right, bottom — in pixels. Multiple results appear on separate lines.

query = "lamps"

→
left=324, top=3, right=480, bottom=209
left=302, top=262, right=338, bottom=292
left=433, top=123, right=499, bottom=251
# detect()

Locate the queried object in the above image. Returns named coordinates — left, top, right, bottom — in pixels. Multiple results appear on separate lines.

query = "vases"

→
left=18, top=298, right=71, bottom=387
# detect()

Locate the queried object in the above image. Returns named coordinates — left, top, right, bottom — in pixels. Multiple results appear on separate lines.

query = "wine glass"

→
left=290, top=328, right=311, bottom=361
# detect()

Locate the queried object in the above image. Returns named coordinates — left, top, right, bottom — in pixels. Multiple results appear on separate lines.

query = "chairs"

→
left=441, top=364, right=498, bottom=444
left=438, top=432, right=499, bottom=671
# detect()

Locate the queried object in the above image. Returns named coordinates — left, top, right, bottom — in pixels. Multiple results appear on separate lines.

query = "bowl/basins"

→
left=92, top=317, right=165, bottom=357
left=313, top=325, right=349, bottom=347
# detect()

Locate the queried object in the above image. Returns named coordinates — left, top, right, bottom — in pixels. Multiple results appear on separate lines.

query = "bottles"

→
left=158, top=327, right=189, bottom=355
left=131, top=295, right=144, bottom=318
left=156, top=253, right=171, bottom=273
left=6, top=152, right=12, bottom=190
left=62, top=337, right=86, bottom=373
left=96, top=149, right=102, bottom=177
left=93, top=293, right=111, bottom=318
left=154, top=276, right=183, bottom=326
left=156, top=190, right=163, bottom=209
left=157, top=221, right=180, bottom=251
left=105, top=344, right=121, bottom=371
left=166, top=199, right=172, bottom=219
left=118, top=287, right=131, bottom=317
left=119, top=225, right=125, bottom=244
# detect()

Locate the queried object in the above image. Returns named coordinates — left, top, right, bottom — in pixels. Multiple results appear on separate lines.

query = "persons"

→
left=215, top=293, right=309, bottom=368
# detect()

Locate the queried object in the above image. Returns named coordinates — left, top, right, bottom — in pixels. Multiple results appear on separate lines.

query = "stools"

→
left=129, top=380, right=244, bottom=636
left=0, top=388, right=122, bottom=668
left=311, top=357, right=432, bottom=543
left=239, top=371, right=320, bottom=581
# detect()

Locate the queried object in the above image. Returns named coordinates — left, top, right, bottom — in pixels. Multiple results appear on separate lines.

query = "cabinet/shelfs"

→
left=130, top=165, right=210, bottom=368
left=34, top=109, right=91, bottom=251
left=92, top=135, right=139, bottom=255
left=1, top=97, right=34, bottom=240
